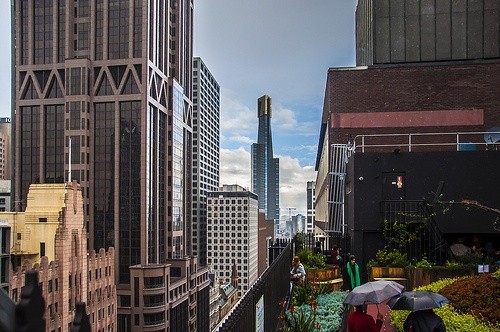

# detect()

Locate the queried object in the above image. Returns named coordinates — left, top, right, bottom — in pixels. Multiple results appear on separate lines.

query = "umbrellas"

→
left=344, top=281, right=405, bottom=311
left=386, top=290, right=449, bottom=312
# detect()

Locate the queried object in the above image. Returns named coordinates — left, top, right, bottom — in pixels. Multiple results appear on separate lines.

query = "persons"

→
left=290, top=256, right=306, bottom=285
left=314, top=241, right=322, bottom=254
left=341, top=255, right=361, bottom=291
left=331, top=245, right=339, bottom=264
left=346, top=303, right=383, bottom=332
left=403, top=309, right=446, bottom=332
